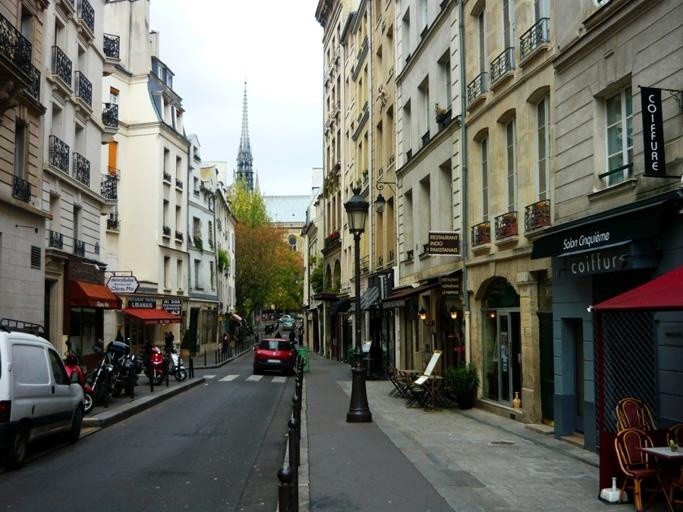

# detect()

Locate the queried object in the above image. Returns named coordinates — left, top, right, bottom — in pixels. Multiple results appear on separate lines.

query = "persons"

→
left=115, top=327, right=174, bottom=353
left=223, top=310, right=304, bottom=353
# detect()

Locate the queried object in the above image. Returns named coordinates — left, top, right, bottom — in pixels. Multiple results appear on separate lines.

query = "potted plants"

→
left=450, top=362, right=480, bottom=409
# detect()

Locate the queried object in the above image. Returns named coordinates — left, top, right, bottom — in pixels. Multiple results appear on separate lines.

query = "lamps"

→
left=419, top=306, right=435, bottom=327
left=449, top=305, right=462, bottom=320
left=374, top=181, right=397, bottom=215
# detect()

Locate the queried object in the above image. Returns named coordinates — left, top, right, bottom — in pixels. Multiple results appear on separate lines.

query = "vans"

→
left=0, top=318, right=85, bottom=466
left=281, top=315, right=290, bottom=322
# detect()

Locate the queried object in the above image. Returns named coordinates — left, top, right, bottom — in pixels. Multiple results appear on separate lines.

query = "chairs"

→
left=389, top=368, right=425, bottom=409
left=667, top=424, right=683, bottom=447
left=615, top=429, right=673, bottom=512
left=616, top=398, right=657, bottom=432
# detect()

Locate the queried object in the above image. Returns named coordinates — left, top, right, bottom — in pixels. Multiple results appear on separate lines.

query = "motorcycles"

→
left=142, top=341, right=165, bottom=386
left=162, top=347, right=186, bottom=382
left=62, top=340, right=95, bottom=413
left=86, top=338, right=142, bottom=406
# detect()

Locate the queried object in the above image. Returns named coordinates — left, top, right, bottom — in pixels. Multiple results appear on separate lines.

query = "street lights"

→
left=344, top=188, right=372, bottom=422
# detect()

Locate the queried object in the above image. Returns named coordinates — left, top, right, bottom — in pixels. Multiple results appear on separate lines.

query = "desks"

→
left=417, top=375, right=445, bottom=407
left=635, top=447, right=683, bottom=512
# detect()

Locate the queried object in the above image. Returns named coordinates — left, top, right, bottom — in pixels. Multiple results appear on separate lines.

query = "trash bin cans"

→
left=295, top=349, right=309, bottom=372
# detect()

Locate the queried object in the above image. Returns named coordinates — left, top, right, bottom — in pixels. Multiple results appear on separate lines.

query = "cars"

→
left=253, top=339, right=298, bottom=374
left=283, top=321, right=292, bottom=330
left=285, top=318, right=295, bottom=327
